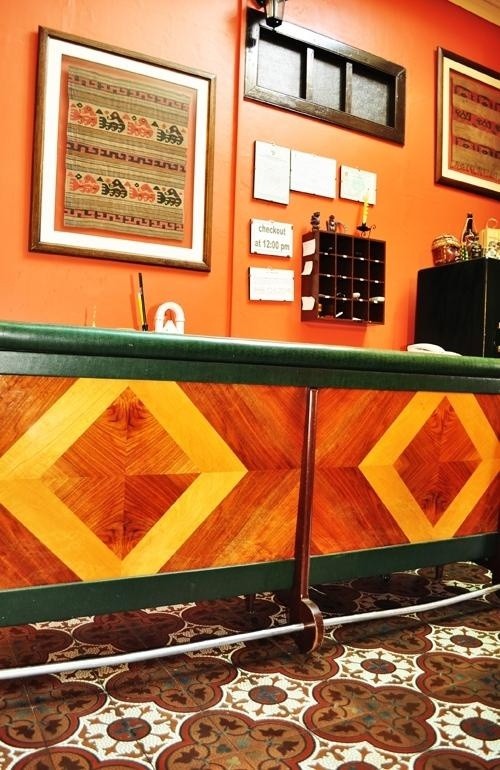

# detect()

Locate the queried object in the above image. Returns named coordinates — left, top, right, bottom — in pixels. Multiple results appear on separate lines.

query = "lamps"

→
left=257, top=0, right=287, bottom=28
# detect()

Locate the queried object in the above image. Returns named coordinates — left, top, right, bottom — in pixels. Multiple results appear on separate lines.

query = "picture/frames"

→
left=434, top=45, right=500, bottom=201
left=29, top=24, right=217, bottom=273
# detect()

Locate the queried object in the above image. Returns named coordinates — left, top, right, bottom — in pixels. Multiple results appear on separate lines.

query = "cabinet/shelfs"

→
left=301, top=231, right=386, bottom=326
left=415, top=258, right=500, bottom=358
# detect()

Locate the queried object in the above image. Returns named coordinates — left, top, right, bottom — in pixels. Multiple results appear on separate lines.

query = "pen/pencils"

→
left=138, top=292, right=143, bottom=324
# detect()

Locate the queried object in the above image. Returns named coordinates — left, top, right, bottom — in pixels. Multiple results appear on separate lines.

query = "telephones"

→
left=407, top=343, right=463, bottom=356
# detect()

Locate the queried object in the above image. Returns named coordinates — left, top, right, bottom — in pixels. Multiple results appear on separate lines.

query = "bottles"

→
left=463, top=212, right=479, bottom=242
